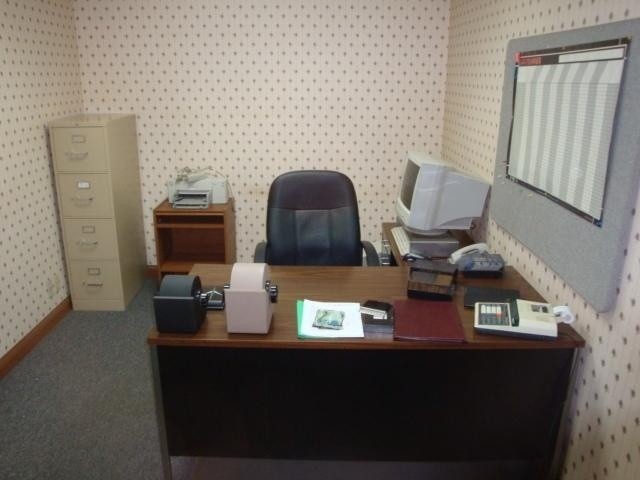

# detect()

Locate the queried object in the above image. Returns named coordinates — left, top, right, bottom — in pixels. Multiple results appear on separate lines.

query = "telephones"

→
left=451, top=243, right=489, bottom=262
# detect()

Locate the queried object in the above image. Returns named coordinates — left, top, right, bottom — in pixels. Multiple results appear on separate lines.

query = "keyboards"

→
left=390, top=226, right=411, bottom=257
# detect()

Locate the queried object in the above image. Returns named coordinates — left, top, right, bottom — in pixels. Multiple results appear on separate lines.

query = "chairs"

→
left=253, top=170, right=380, bottom=266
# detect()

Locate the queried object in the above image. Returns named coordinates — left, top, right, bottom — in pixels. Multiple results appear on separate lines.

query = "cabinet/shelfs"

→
left=152, top=197, right=236, bottom=290
left=381, top=222, right=475, bottom=266
left=49, top=113, right=148, bottom=311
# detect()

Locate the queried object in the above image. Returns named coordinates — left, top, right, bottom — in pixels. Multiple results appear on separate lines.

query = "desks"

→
left=145, top=265, right=585, bottom=479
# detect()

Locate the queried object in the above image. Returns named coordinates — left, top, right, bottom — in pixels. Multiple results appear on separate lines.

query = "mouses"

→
left=403, top=253, right=426, bottom=262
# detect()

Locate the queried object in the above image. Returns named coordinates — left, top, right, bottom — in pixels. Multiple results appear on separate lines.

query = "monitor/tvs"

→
left=395, top=150, right=491, bottom=237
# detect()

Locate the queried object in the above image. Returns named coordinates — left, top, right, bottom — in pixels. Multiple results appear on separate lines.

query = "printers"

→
left=167, top=166, right=238, bottom=209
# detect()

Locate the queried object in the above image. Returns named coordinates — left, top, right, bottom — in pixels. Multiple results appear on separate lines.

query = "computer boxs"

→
left=396, top=216, right=459, bottom=259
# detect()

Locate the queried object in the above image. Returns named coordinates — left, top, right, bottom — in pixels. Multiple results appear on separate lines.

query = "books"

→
left=295, top=294, right=395, bottom=342
